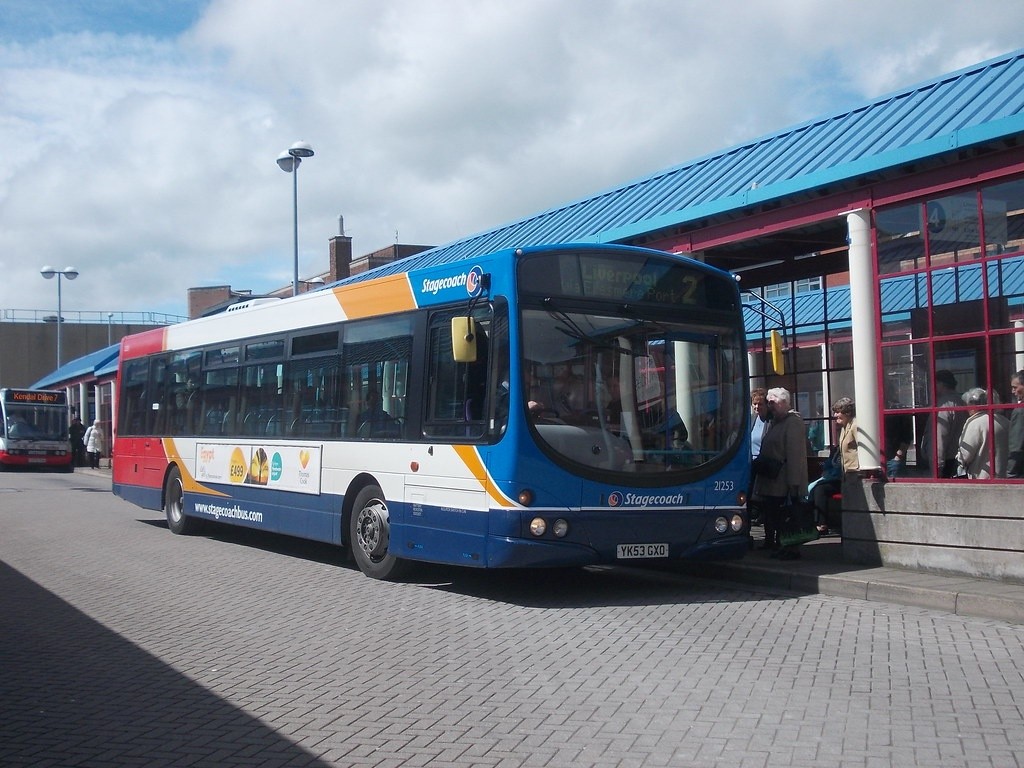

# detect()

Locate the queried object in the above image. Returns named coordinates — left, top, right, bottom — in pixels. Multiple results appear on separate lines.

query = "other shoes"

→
left=71, top=463, right=81, bottom=468
left=761, top=536, right=782, bottom=546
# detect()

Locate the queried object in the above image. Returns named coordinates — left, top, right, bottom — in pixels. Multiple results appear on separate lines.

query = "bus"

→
left=111, top=242, right=793, bottom=581
left=0, top=387, right=74, bottom=474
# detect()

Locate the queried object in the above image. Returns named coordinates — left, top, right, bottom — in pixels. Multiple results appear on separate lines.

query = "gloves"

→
left=886, top=459, right=902, bottom=477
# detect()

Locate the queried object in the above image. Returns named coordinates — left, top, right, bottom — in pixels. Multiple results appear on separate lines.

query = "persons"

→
left=69, top=418, right=104, bottom=469
left=606, top=369, right=622, bottom=409
left=182, top=374, right=200, bottom=403
left=886, top=370, right=1024, bottom=479
left=809, top=398, right=858, bottom=533
left=359, top=391, right=391, bottom=427
left=539, top=351, right=584, bottom=411
left=750, top=387, right=808, bottom=561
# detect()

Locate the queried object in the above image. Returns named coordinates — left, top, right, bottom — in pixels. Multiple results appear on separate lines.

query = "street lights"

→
left=40, top=264, right=80, bottom=374
left=297, top=229, right=300, bottom=233
left=108, top=313, right=113, bottom=347
left=275, top=139, right=315, bottom=296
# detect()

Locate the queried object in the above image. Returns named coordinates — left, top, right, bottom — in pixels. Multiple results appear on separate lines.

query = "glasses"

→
left=766, top=400, right=779, bottom=404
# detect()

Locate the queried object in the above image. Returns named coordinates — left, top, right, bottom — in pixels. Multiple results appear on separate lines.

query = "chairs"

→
left=121, top=380, right=488, bottom=441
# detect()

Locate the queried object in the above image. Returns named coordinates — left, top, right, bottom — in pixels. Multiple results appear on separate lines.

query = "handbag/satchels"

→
left=773, top=487, right=829, bottom=546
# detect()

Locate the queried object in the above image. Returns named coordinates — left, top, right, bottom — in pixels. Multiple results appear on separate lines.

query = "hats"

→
left=75, top=418, right=81, bottom=420
